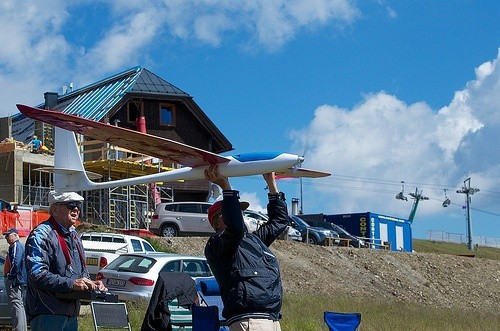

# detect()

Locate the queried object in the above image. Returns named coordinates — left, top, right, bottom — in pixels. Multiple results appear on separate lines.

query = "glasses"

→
left=6, top=234, right=13, bottom=237
left=59, top=202, right=82, bottom=210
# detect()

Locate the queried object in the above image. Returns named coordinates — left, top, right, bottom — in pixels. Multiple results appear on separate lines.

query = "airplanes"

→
left=14, top=102, right=332, bottom=193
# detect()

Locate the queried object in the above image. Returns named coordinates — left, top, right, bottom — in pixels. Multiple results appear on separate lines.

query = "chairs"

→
left=176, top=262, right=197, bottom=271
left=194, top=278, right=230, bottom=331
left=324, top=311, right=361, bottom=331
left=90, top=302, right=131, bottom=331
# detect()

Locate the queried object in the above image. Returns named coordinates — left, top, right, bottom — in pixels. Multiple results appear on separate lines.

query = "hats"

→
left=48, top=189, right=84, bottom=205
left=3, top=228, right=19, bottom=235
left=208, top=201, right=249, bottom=227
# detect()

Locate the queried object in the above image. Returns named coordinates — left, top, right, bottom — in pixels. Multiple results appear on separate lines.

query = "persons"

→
left=2, top=228, right=27, bottom=331
left=25, top=192, right=108, bottom=331
left=204, top=164, right=289, bottom=331
left=30, top=135, right=51, bottom=154
left=9, top=205, right=23, bottom=227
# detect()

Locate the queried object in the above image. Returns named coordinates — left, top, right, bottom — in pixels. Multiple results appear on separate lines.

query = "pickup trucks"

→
left=79, top=233, right=157, bottom=281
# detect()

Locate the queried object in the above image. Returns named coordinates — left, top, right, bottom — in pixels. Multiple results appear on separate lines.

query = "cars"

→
left=95, top=251, right=213, bottom=301
left=243, top=209, right=303, bottom=242
left=0, top=255, right=27, bottom=322
left=303, top=219, right=367, bottom=248
left=264, top=213, right=340, bottom=246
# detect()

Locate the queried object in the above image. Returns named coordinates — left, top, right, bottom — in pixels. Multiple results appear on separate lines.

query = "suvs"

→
left=149, top=201, right=257, bottom=238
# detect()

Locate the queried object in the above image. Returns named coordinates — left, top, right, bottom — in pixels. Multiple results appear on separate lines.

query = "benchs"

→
left=366, top=242, right=389, bottom=250
left=325, top=237, right=352, bottom=247
